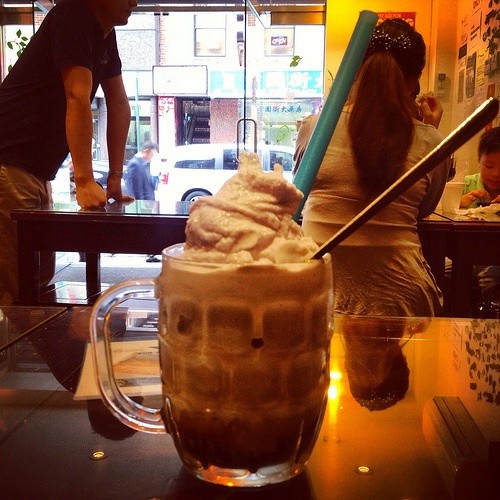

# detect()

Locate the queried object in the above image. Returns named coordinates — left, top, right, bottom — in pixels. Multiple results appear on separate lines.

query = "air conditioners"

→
left=422, top=396, right=490, bottom=500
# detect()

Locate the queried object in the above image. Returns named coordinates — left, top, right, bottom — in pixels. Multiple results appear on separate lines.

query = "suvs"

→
left=157, top=142, right=295, bottom=215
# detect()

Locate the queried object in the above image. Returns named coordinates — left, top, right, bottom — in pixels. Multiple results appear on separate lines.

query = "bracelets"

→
left=108, top=170, right=123, bottom=178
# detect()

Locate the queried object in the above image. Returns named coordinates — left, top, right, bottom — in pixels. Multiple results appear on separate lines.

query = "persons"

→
left=339, top=316, right=411, bottom=412
left=0, top=305, right=144, bottom=441
left=0, top=0, right=137, bottom=289
left=293, top=17, right=450, bottom=316
left=445, top=127, right=500, bottom=298
left=124, top=143, right=165, bottom=201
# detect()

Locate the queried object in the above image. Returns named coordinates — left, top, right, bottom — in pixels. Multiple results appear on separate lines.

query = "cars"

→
left=62, top=157, right=128, bottom=193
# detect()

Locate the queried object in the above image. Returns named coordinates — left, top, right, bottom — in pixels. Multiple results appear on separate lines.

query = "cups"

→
left=89, top=242, right=335, bottom=488
left=442, top=181, right=465, bottom=215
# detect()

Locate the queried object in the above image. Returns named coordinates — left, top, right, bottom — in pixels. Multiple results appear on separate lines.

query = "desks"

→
left=0, top=306, right=500, bottom=500
left=8, top=199, right=500, bottom=318
